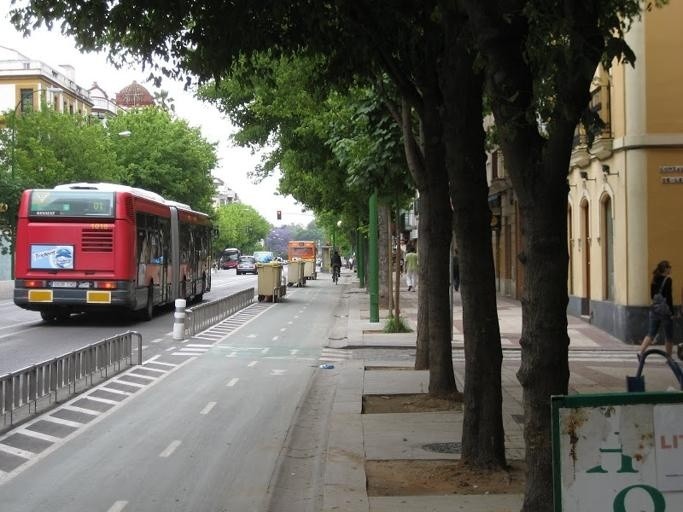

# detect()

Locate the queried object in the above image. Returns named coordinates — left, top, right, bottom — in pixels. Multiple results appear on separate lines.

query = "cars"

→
left=236, top=256, right=257, bottom=275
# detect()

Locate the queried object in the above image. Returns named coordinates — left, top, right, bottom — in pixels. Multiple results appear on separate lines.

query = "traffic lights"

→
left=277, top=211, right=281, bottom=219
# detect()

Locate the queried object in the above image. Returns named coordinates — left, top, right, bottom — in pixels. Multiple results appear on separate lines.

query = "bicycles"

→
left=333, top=265, right=339, bottom=285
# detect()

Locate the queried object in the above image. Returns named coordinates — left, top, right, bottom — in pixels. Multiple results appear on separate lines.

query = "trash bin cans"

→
left=288, top=261, right=305, bottom=287
left=301, top=259, right=317, bottom=280
left=255, top=262, right=286, bottom=301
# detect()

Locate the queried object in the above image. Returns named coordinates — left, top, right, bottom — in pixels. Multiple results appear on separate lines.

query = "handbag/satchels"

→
left=650, top=276, right=672, bottom=319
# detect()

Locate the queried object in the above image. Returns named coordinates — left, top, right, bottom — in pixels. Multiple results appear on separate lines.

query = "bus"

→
left=13, top=182, right=220, bottom=321
left=222, top=248, right=241, bottom=270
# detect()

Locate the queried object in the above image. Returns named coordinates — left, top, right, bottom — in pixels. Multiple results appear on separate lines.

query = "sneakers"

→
left=636, top=351, right=646, bottom=364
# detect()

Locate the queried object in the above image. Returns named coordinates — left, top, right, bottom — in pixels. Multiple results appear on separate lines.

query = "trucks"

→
left=288, top=241, right=318, bottom=261
left=253, top=251, right=273, bottom=263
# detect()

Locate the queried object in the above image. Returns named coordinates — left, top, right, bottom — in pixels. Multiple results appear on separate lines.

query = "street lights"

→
left=10, top=88, right=63, bottom=280
left=102, top=131, right=131, bottom=142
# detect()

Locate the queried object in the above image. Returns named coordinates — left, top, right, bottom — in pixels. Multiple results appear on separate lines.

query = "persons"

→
left=403, top=246, right=418, bottom=292
left=329, top=251, right=341, bottom=283
left=451, top=248, right=461, bottom=291
left=635, top=259, right=678, bottom=366
